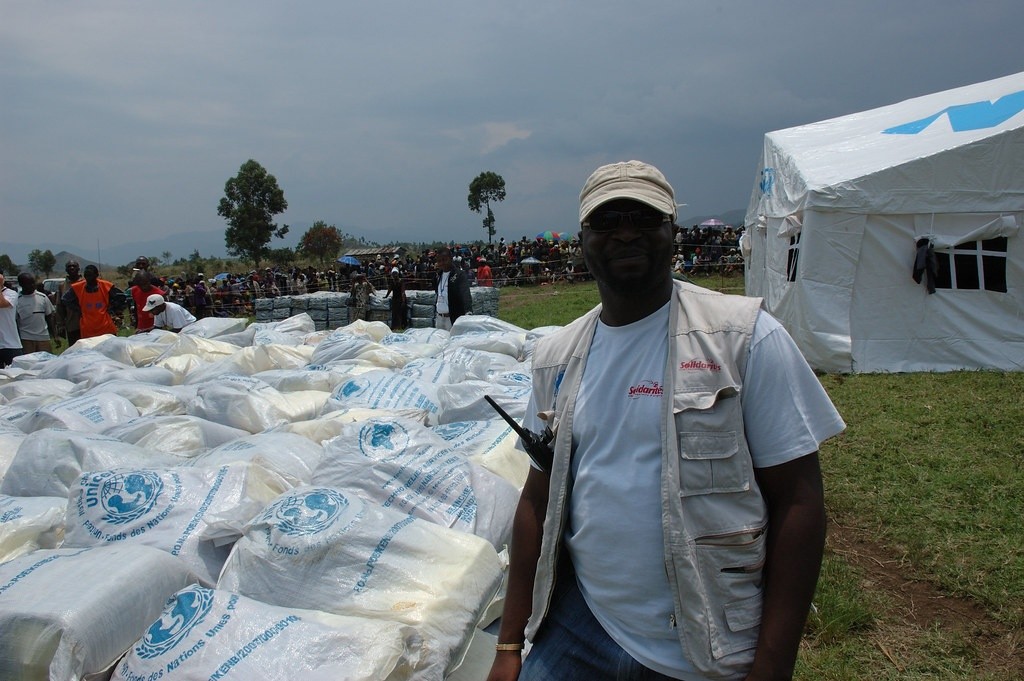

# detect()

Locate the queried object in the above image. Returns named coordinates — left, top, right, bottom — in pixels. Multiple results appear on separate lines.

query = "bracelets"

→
left=496, top=644, right=524, bottom=651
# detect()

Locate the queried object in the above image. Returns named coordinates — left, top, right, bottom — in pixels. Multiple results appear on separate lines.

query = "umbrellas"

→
left=536, top=230, right=560, bottom=240
left=700, top=218, right=725, bottom=226
left=558, top=232, right=575, bottom=240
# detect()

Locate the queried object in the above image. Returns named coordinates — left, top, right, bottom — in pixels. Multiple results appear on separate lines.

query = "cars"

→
left=42, top=278, right=67, bottom=296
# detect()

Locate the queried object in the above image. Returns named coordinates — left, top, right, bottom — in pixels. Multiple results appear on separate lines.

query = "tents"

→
left=739, top=71, right=1024, bottom=374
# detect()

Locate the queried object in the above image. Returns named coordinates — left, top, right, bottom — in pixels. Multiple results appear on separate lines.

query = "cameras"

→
left=133, top=269, right=140, bottom=272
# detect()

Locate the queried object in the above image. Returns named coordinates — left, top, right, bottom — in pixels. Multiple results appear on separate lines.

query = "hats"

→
left=199, top=281, right=204, bottom=284
left=142, top=294, right=164, bottom=312
left=198, top=273, right=204, bottom=277
left=579, top=160, right=677, bottom=229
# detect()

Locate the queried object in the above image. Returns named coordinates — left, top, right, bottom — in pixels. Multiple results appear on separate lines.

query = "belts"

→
left=439, top=313, right=450, bottom=317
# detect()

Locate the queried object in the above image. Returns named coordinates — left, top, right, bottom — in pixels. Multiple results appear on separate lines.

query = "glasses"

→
left=582, top=208, right=671, bottom=233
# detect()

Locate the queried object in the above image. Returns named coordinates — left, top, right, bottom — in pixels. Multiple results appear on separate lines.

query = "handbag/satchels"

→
left=345, top=289, right=358, bottom=307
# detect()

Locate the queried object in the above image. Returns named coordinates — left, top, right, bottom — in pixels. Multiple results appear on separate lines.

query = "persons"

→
left=486, top=160, right=847, bottom=680
left=0, top=224, right=747, bottom=370
